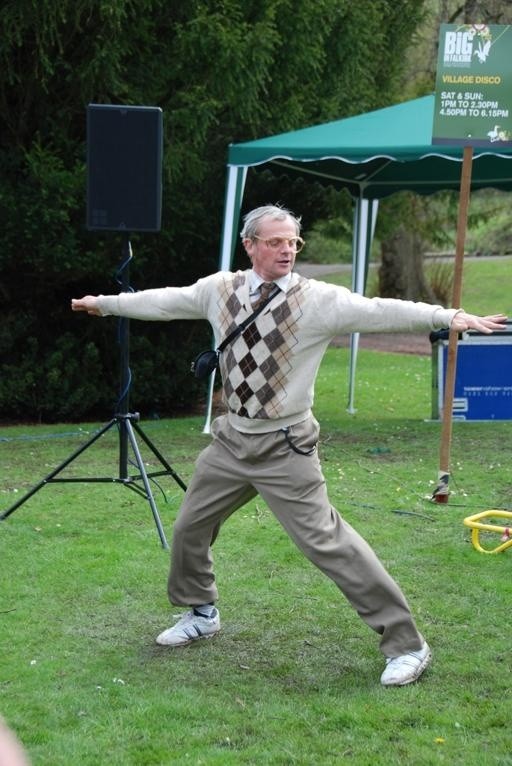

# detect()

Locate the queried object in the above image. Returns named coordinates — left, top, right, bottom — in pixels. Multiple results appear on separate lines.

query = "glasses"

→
left=253, top=235, right=305, bottom=253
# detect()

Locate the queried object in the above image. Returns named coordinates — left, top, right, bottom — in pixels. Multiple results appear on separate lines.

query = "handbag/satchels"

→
left=191, top=349, right=223, bottom=392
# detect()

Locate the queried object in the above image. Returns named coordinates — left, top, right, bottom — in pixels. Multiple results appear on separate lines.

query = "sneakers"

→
left=381, top=640, right=431, bottom=685
left=156, top=607, right=220, bottom=646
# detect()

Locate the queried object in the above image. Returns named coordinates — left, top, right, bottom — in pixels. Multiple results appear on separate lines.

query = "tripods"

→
left=0, top=236, right=189, bottom=550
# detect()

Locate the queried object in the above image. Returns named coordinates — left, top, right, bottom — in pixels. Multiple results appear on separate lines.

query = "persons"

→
left=70, top=204, right=510, bottom=689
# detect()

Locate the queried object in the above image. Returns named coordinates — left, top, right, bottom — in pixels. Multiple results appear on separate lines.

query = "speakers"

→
left=85, top=104, right=163, bottom=234
